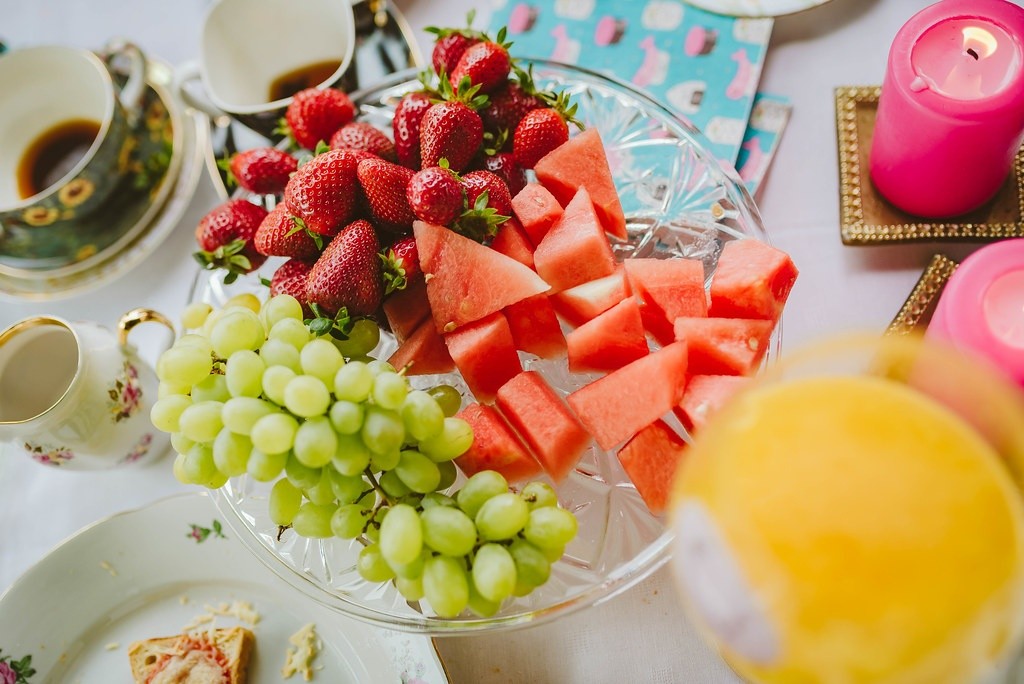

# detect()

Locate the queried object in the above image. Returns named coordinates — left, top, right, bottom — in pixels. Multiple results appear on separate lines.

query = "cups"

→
left=196, top=0, right=357, bottom=118
left=0, top=39, right=151, bottom=232
left=660, top=333, right=1024, bottom=683
left=1, top=306, right=178, bottom=478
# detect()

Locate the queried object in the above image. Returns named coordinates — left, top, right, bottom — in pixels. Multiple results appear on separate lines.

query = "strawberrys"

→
left=191, top=10, right=586, bottom=340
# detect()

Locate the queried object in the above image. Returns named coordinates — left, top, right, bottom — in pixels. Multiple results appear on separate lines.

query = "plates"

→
left=2, top=488, right=454, bottom=682
left=1, top=65, right=186, bottom=279
left=184, top=56, right=788, bottom=635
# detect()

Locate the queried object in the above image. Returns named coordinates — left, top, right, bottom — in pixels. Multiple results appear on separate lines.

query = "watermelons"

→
left=388, top=128, right=797, bottom=517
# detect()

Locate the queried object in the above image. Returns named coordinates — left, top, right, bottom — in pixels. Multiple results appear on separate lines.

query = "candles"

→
left=868, top=0, right=1024, bottom=217
left=923, top=239, right=1024, bottom=395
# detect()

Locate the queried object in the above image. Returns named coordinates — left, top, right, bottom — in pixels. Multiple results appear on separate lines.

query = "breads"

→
left=128, top=627, right=255, bottom=684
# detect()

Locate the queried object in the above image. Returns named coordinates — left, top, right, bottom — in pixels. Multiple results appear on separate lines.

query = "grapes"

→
left=151, top=294, right=580, bottom=620
